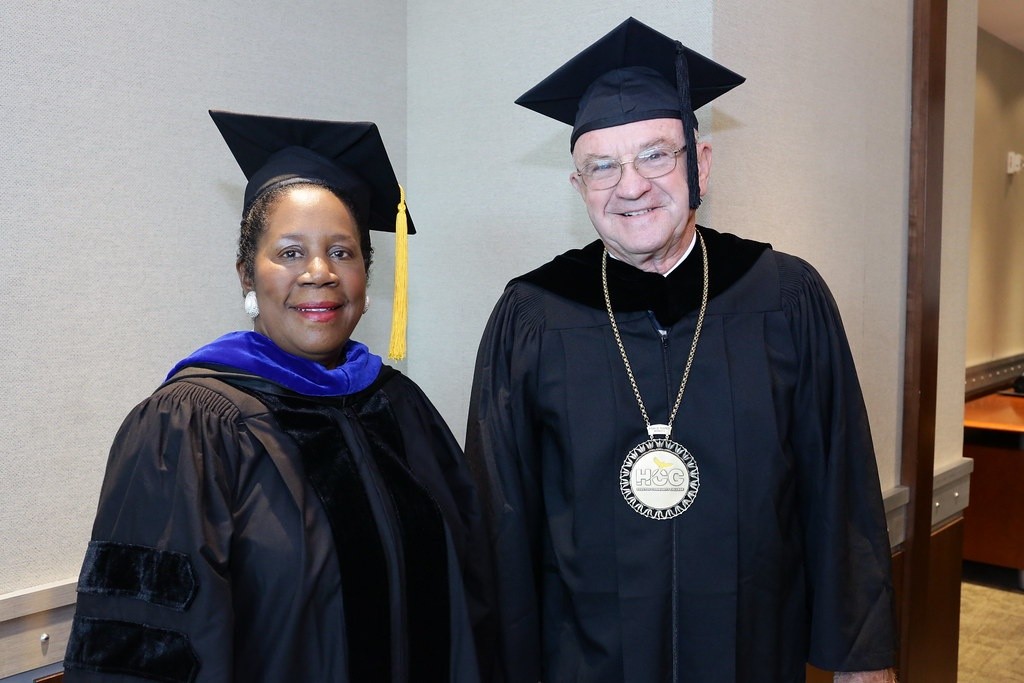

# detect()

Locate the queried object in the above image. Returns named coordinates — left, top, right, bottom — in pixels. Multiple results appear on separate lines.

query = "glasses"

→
left=575, top=144, right=688, bottom=191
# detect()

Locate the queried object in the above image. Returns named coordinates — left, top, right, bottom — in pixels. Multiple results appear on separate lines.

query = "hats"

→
left=512, top=17, right=747, bottom=206
left=208, top=109, right=416, bottom=362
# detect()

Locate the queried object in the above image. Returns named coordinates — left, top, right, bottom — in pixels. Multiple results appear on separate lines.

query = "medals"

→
left=617, top=437, right=701, bottom=521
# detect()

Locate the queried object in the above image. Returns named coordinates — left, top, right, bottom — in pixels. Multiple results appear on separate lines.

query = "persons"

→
left=63, top=109, right=464, bottom=682
left=465, top=15, right=900, bottom=682
